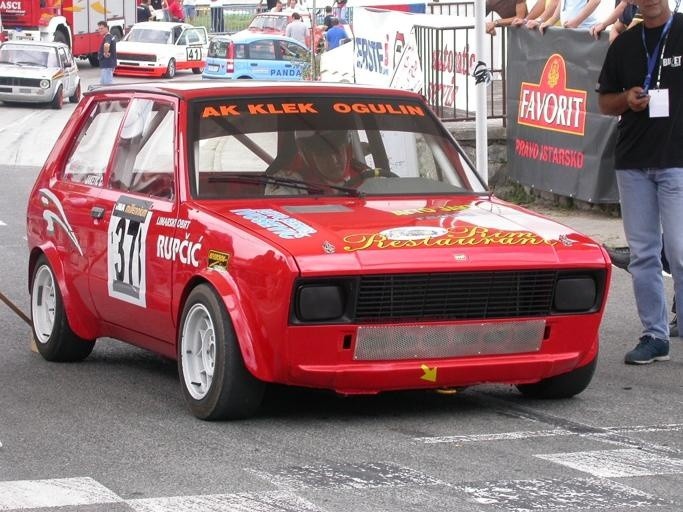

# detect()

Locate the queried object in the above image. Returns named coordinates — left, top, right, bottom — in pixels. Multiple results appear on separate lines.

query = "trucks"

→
left=0, top=1, right=136, bottom=65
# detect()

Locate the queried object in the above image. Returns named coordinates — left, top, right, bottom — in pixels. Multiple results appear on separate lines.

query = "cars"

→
left=0, top=43, right=80, bottom=108
left=26, top=79, right=612, bottom=421
left=202, top=11, right=343, bottom=80
left=113, top=20, right=208, bottom=78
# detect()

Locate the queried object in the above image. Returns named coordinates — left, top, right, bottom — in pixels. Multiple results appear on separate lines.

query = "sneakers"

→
left=625, top=336, right=671, bottom=364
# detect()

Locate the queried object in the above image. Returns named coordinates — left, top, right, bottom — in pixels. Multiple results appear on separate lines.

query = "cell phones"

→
left=636, top=92, right=646, bottom=99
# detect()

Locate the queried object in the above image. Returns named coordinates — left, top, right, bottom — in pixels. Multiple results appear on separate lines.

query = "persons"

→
left=595, top=0, right=683, bottom=363
left=97, top=21, right=117, bottom=85
left=603, top=233, right=683, bottom=337
left=264, top=130, right=378, bottom=196
left=484, top=0, right=683, bottom=44
left=258, top=0, right=350, bottom=51
left=136, top=0, right=197, bottom=24
left=209, top=0, right=224, bottom=32
